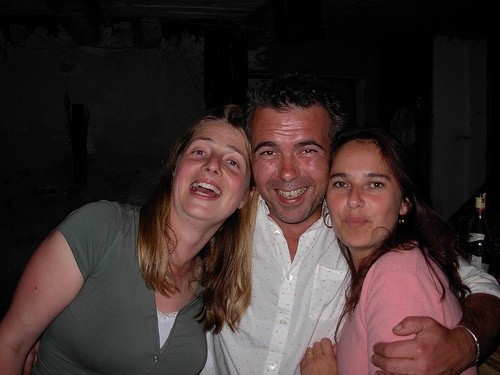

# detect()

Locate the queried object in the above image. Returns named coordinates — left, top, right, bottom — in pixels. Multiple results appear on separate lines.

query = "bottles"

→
left=463, top=191, right=493, bottom=273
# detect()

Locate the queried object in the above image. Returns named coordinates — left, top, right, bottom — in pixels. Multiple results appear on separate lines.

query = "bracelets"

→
left=454, top=323, right=482, bottom=366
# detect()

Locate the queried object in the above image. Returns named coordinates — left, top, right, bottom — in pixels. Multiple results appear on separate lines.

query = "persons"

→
left=301, top=122, right=478, bottom=374
left=26, top=73, right=500, bottom=375
left=1, top=104, right=262, bottom=375
